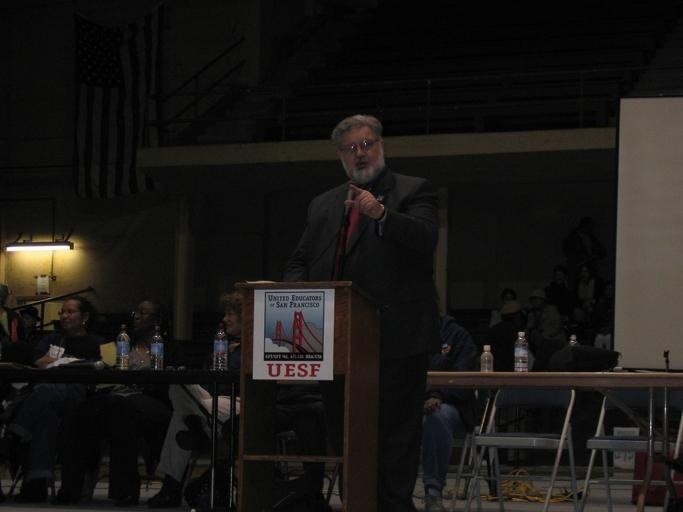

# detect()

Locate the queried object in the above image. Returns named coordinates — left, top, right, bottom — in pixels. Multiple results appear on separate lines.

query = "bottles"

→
left=117, top=325, right=131, bottom=369
left=214, top=324, right=228, bottom=370
left=150, top=327, right=164, bottom=369
left=514, top=332, right=528, bottom=372
left=481, top=345, right=493, bottom=372
left=570, top=335, right=578, bottom=345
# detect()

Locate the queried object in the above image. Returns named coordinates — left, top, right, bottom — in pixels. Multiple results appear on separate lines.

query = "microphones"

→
left=331, top=181, right=355, bottom=281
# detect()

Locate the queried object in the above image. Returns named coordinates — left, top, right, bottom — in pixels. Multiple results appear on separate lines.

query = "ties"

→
left=344, top=208, right=360, bottom=249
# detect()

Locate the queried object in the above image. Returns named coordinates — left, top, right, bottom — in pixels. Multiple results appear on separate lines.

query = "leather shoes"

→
left=20, top=473, right=58, bottom=500
left=148, top=484, right=177, bottom=508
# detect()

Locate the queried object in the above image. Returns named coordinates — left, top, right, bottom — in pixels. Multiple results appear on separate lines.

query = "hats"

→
left=529, top=290, right=546, bottom=300
left=500, top=301, right=520, bottom=315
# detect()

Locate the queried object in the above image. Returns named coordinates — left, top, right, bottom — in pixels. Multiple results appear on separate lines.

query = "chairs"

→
left=449, top=309, right=496, bottom=337
left=276, top=402, right=341, bottom=504
left=579, top=367, right=683, bottom=512
left=465, top=389, right=578, bottom=512
left=451, top=389, right=490, bottom=512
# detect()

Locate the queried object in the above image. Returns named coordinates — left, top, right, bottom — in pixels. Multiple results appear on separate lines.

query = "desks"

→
left=0, top=368, right=223, bottom=512
left=234, top=281, right=381, bottom=512
left=224, top=370, right=683, bottom=512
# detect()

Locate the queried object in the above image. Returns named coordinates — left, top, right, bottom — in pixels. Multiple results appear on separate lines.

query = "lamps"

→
left=5, top=242, right=73, bottom=253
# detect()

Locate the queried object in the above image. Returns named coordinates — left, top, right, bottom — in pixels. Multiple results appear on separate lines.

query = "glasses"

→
left=132, top=310, right=151, bottom=318
left=58, top=307, right=75, bottom=314
left=338, top=137, right=379, bottom=155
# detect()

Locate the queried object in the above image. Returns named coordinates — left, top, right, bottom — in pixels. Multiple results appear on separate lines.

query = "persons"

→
left=0, top=296, right=103, bottom=503
left=147, top=291, right=241, bottom=508
left=578, top=216, right=612, bottom=277
left=564, top=308, right=593, bottom=346
left=17, top=304, right=49, bottom=364
left=0, top=283, right=12, bottom=357
left=545, top=265, right=580, bottom=311
left=420, top=315, right=477, bottom=512
left=282, top=114, right=440, bottom=512
left=524, top=286, right=567, bottom=355
left=490, top=288, right=526, bottom=329
left=594, top=326, right=611, bottom=350
left=500, top=300, right=523, bottom=321
left=578, top=265, right=610, bottom=306
left=92, top=299, right=175, bottom=506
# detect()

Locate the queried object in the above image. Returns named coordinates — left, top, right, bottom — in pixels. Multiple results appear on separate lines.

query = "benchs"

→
left=289, top=66, right=632, bottom=97
left=270, top=81, right=620, bottom=116
left=283, top=100, right=610, bottom=138
left=308, top=48, right=645, bottom=78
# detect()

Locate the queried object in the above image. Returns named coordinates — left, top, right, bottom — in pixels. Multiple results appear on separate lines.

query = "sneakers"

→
left=422, top=484, right=443, bottom=511
